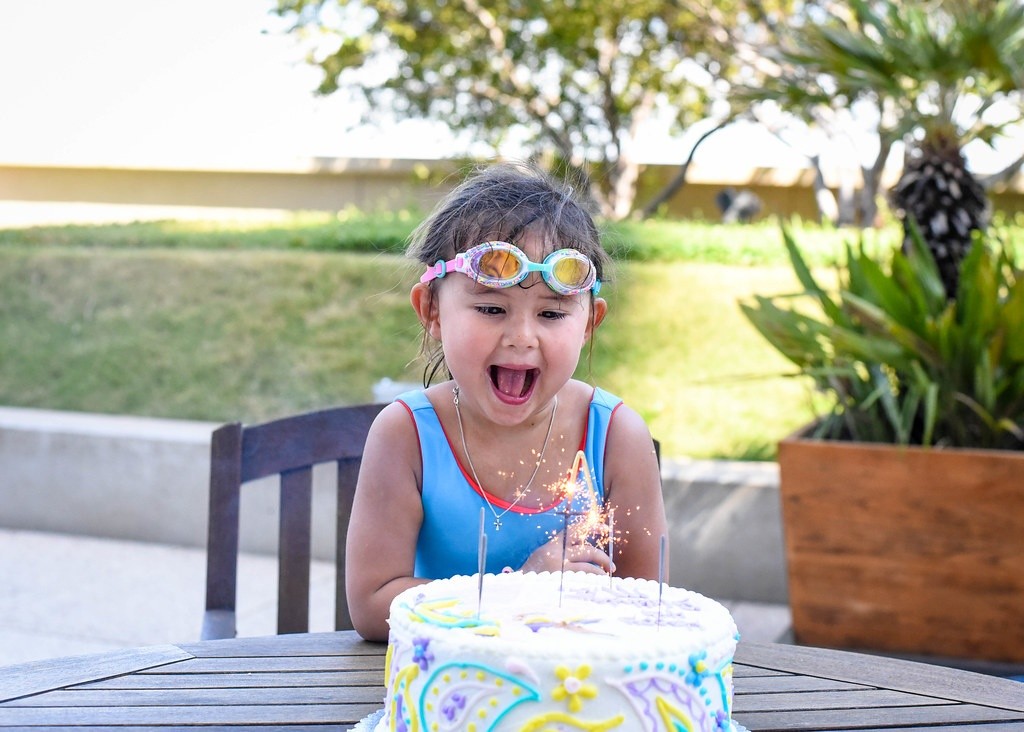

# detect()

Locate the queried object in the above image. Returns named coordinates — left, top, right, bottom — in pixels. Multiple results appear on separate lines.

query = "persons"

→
left=343, top=161, right=669, bottom=641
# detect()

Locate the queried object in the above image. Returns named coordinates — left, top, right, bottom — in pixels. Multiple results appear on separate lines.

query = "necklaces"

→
left=452, top=385, right=558, bottom=531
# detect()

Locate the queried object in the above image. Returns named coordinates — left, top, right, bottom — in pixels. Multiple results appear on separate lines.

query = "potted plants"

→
left=730, top=209, right=1024, bottom=669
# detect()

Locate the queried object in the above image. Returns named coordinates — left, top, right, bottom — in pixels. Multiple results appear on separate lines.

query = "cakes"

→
left=369, top=568, right=740, bottom=732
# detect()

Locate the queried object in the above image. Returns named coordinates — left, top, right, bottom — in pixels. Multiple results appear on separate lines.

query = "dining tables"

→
left=0, top=632, right=1024, bottom=731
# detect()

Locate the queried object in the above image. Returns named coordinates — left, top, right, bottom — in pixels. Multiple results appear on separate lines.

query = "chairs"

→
left=206, top=404, right=659, bottom=632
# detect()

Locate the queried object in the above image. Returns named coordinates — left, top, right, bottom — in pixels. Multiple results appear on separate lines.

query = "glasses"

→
left=420, top=241, right=601, bottom=296
